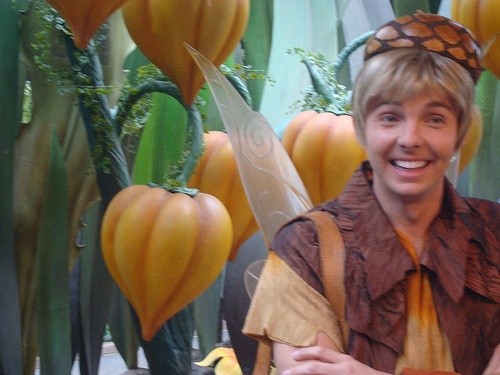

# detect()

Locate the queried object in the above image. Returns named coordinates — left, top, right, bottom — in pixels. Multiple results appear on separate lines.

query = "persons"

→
left=242, top=14, right=500, bottom=375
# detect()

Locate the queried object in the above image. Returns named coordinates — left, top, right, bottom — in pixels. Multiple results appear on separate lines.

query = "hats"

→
left=364, top=11, right=487, bottom=85
